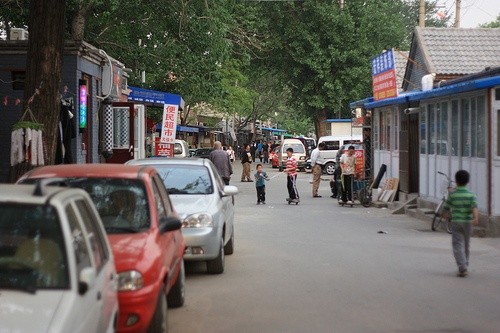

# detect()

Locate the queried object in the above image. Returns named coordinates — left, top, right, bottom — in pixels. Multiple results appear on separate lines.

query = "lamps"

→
left=79, top=79, right=88, bottom=133
left=102, top=98, right=113, bottom=158
left=113, top=108, right=128, bottom=147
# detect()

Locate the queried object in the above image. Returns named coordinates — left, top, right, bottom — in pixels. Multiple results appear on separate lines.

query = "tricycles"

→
left=335, top=168, right=372, bottom=207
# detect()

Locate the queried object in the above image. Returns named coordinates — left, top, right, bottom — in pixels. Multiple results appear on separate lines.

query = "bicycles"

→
left=430, top=171, right=454, bottom=234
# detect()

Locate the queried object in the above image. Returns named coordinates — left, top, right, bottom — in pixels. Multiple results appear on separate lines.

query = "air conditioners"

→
left=10, top=28, right=29, bottom=40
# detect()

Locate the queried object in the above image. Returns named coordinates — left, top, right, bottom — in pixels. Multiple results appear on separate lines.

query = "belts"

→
left=315, top=163, right=322, bottom=167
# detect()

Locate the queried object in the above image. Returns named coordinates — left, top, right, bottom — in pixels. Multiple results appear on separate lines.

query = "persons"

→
left=330, top=145, right=345, bottom=198
left=339, top=146, right=356, bottom=203
left=441, top=170, right=479, bottom=277
left=210, top=141, right=233, bottom=185
left=251, top=141, right=279, bottom=163
left=311, top=143, right=324, bottom=197
left=241, top=146, right=253, bottom=182
left=285, top=148, right=299, bottom=201
left=227, top=146, right=235, bottom=162
left=254, top=164, right=270, bottom=204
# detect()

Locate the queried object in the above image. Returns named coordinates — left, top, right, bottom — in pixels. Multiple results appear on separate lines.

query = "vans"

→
left=173, top=139, right=191, bottom=159
left=311, top=135, right=363, bottom=175
left=271, top=138, right=307, bottom=172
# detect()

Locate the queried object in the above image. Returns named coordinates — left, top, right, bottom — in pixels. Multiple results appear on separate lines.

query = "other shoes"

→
left=256, top=202, right=260, bottom=205
left=262, top=201, right=266, bottom=204
left=338, top=199, right=354, bottom=204
left=313, top=194, right=322, bottom=197
left=286, top=198, right=299, bottom=202
left=241, top=179, right=253, bottom=182
left=457, top=271, right=468, bottom=277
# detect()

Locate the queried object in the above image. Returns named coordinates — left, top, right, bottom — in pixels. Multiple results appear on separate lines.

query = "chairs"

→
left=17, top=239, right=60, bottom=287
left=109, top=191, right=138, bottom=222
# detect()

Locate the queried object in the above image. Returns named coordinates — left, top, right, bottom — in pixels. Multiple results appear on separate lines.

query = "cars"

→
left=101, top=155, right=239, bottom=274
left=0, top=177, right=122, bottom=333
left=189, top=147, right=213, bottom=158
left=12, top=162, right=186, bottom=333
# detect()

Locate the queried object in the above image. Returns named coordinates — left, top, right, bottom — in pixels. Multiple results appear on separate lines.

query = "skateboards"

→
left=340, top=201, right=363, bottom=207
left=286, top=199, right=299, bottom=205
